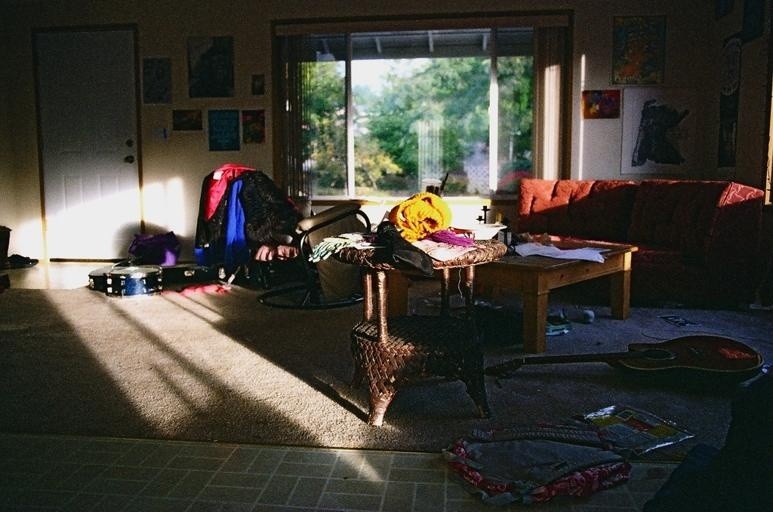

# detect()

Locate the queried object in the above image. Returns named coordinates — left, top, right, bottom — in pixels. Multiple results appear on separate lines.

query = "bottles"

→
left=493, top=212, right=513, bottom=245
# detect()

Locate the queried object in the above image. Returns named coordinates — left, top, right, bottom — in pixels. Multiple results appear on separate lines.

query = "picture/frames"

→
left=609, top=12, right=698, bottom=176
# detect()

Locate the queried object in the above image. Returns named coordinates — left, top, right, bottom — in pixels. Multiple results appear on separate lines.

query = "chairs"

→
left=199, top=164, right=378, bottom=310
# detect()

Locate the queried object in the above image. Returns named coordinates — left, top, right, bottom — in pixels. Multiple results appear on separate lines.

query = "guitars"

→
left=485, top=336, right=764, bottom=382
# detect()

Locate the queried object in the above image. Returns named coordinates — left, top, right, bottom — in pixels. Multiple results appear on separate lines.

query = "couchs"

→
left=517, top=179, right=764, bottom=301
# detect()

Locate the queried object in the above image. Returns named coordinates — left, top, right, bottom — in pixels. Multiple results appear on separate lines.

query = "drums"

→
left=88, top=265, right=164, bottom=296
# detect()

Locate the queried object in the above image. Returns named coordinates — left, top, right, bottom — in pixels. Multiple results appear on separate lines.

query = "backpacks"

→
left=442, top=419, right=635, bottom=505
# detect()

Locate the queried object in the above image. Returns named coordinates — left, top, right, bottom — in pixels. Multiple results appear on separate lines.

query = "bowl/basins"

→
left=455, top=223, right=508, bottom=241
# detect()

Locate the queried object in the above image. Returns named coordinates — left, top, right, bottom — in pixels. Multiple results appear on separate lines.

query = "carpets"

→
left=1, top=287, right=772, bottom=456
